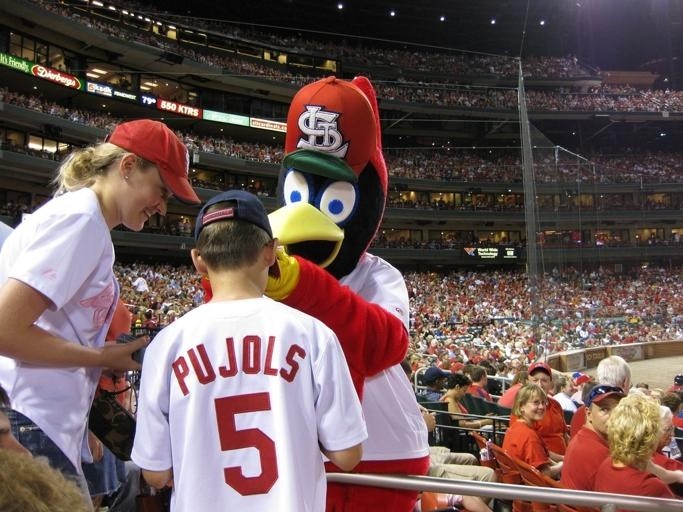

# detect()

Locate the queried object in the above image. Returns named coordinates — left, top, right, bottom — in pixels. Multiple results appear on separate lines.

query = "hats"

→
left=107, top=117, right=201, bottom=205
left=194, top=189, right=274, bottom=243
left=584, top=384, right=627, bottom=409
left=575, top=375, right=593, bottom=386
left=421, top=367, right=451, bottom=385
left=528, top=362, right=553, bottom=377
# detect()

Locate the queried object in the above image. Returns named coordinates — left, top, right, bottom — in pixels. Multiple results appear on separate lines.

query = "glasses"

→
left=524, top=399, right=549, bottom=406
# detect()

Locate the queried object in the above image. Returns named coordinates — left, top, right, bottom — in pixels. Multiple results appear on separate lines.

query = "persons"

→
left=2, top=2, right=682, bottom=509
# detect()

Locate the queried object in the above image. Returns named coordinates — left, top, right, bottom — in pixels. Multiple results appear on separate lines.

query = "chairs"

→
left=414, top=395, right=473, bottom=451
left=563, top=411, right=573, bottom=425
left=461, top=394, right=513, bottom=429
left=472, top=430, right=602, bottom=511
